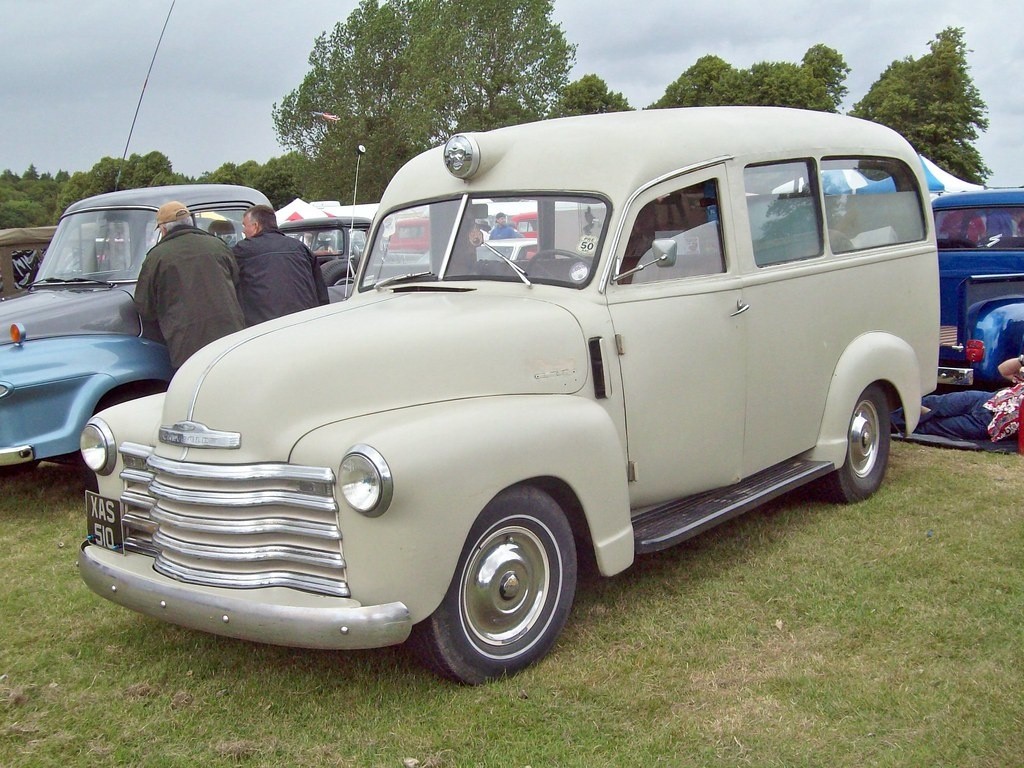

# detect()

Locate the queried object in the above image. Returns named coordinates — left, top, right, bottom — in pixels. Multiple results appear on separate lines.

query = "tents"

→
left=772, top=153, right=986, bottom=196
left=275, top=196, right=341, bottom=238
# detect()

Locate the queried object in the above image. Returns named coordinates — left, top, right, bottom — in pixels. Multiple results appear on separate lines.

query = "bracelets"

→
left=1018, top=355, right=1024, bottom=366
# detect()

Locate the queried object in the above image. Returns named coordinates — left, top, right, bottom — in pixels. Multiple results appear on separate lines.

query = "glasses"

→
left=242, top=221, right=257, bottom=228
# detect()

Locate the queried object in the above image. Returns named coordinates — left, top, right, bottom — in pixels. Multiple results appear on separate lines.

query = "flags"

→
left=311, top=110, right=342, bottom=125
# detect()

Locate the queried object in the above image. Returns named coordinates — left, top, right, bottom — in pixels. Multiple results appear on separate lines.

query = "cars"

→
left=80, top=106, right=943, bottom=690
left=929, top=189, right=1023, bottom=393
left=470, top=195, right=538, bottom=265
left=276, top=215, right=378, bottom=289
left=0, top=184, right=275, bottom=493
left=0, top=224, right=125, bottom=300
left=387, top=215, right=431, bottom=263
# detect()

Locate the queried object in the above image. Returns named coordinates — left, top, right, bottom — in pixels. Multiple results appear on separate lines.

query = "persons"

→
left=890, top=352, right=1024, bottom=459
left=229, top=202, right=330, bottom=326
left=134, top=200, right=247, bottom=370
left=487, top=212, right=525, bottom=240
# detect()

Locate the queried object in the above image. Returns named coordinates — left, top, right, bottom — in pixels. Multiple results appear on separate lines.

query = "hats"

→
left=495, top=213, right=506, bottom=224
left=154, top=201, right=191, bottom=231
